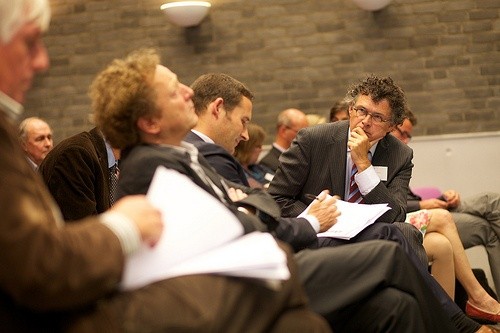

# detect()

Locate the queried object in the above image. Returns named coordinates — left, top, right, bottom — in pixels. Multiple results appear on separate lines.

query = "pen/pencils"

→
left=304, top=193, right=320, bottom=200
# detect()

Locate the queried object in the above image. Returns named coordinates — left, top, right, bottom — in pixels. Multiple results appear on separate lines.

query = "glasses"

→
left=396, top=127, right=411, bottom=140
left=354, top=107, right=391, bottom=123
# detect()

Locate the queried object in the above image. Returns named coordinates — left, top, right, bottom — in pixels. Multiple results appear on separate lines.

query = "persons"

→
left=17, top=118, right=54, bottom=169
left=0, top=0, right=330, bottom=333
left=90, top=49, right=457, bottom=333
left=233, top=101, right=350, bottom=196
left=183, top=71, right=500, bottom=333
left=385, top=105, right=500, bottom=323
left=267, top=75, right=430, bottom=278
left=36, top=126, right=121, bottom=226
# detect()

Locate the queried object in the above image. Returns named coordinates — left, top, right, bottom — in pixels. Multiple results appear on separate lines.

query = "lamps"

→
left=160, top=1, right=211, bottom=28
left=353, top=0, right=392, bottom=10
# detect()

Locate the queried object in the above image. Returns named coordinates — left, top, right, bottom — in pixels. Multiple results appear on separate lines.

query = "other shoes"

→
left=465, top=301, right=500, bottom=322
left=475, top=325, right=500, bottom=333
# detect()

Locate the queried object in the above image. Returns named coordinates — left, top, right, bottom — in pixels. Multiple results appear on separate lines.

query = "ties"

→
left=109, top=165, right=119, bottom=208
left=348, top=163, right=365, bottom=204
